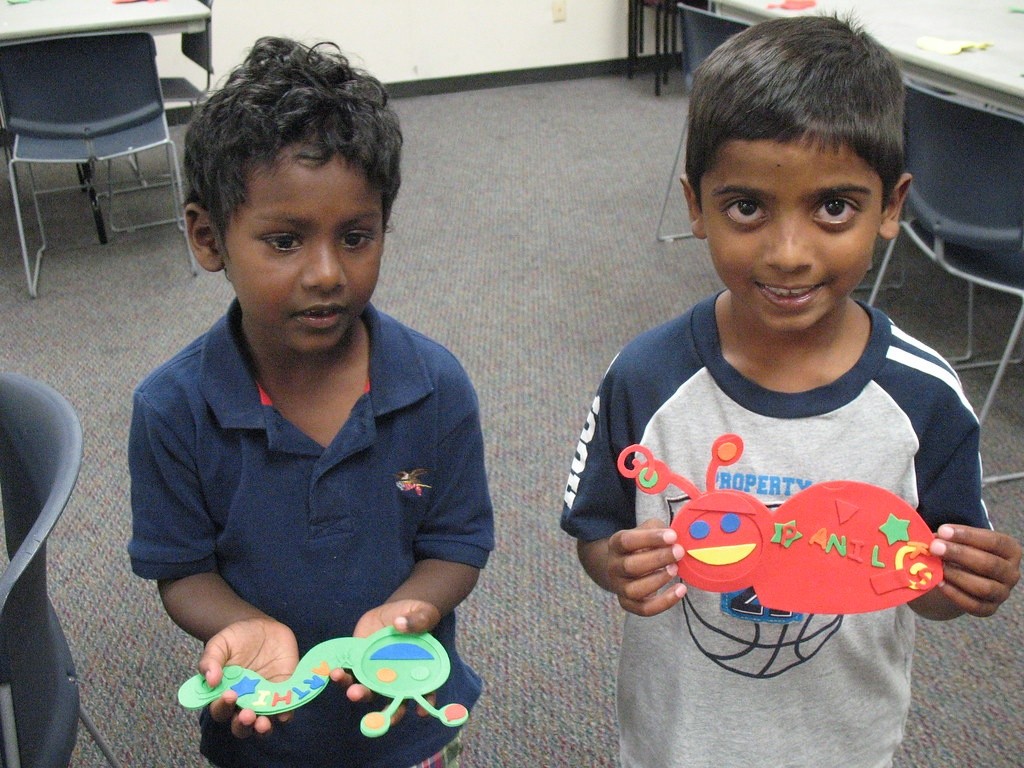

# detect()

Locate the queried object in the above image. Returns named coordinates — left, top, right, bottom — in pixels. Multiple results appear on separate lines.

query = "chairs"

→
left=868, top=77, right=1024, bottom=487
left=626, top=1, right=751, bottom=241
left=0, top=33, right=199, bottom=308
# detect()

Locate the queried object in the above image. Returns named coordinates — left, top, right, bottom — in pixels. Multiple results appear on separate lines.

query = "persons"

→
left=560, top=17, right=1024, bottom=768
left=126, top=35, right=496, bottom=768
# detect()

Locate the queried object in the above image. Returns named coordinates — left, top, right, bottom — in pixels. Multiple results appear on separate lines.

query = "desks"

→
left=686, top=0, right=1024, bottom=88
left=0, top=0, right=213, bottom=113
